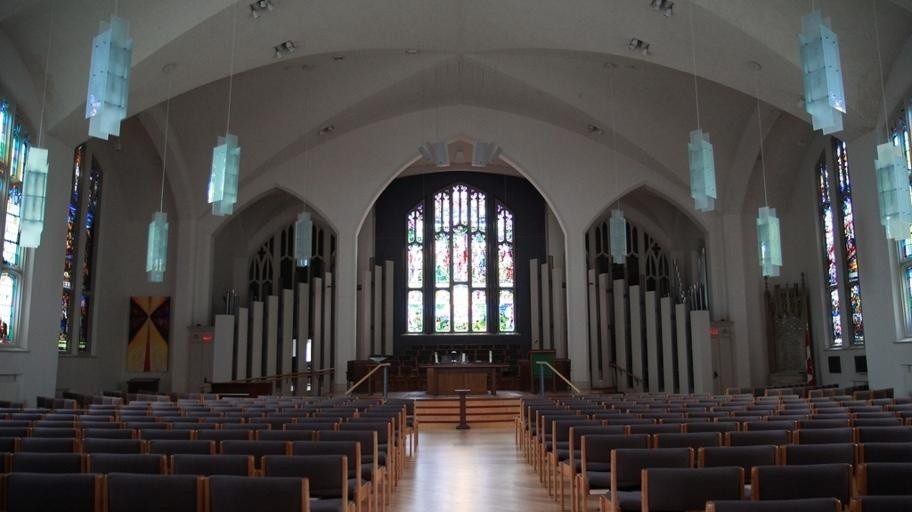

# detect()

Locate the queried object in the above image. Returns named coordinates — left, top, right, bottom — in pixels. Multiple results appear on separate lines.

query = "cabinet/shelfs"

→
left=347, top=343, right=571, bottom=391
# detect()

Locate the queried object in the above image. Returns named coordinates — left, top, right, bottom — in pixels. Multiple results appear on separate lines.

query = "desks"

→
left=417, top=361, right=510, bottom=395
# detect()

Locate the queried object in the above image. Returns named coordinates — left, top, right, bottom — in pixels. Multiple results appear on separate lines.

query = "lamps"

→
left=293, top=63, right=313, bottom=269
left=689, top=2, right=718, bottom=212
left=798, top=0, right=849, bottom=136
left=18, top=13, right=55, bottom=246
left=605, top=61, right=627, bottom=267
left=86, top=1, right=134, bottom=138
left=208, top=2, right=242, bottom=216
left=872, top=2, right=911, bottom=242
left=146, top=61, right=177, bottom=283
left=745, top=62, right=784, bottom=278
